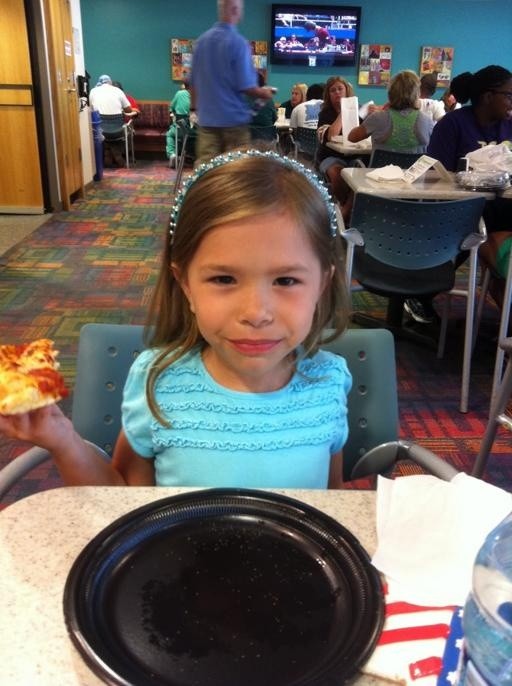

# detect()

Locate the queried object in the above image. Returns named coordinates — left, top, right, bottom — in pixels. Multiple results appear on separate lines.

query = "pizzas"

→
left=0, top=337, right=69, bottom=416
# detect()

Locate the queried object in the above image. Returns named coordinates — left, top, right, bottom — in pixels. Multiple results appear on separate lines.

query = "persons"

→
left=0, top=142, right=352, bottom=490
left=249, top=73, right=279, bottom=144
left=381, top=47, right=390, bottom=58
left=188, top=0, right=273, bottom=173
left=89, top=75, right=132, bottom=168
left=166, top=78, right=197, bottom=168
left=274, top=20, right=353, bottom=54
left=113, top=81, right=141, bottom=123
left=277, top=65, right=512, bottom=326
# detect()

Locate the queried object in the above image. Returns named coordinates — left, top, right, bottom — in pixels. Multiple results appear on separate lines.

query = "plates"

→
left=60, top=486, right=387, bottom=686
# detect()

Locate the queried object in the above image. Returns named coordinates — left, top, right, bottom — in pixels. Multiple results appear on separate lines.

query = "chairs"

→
left=172, top=116, right=511, bottom=195
left=340, top=193, right=489, bottom=414
left=0, top=318, right=458, bottom=486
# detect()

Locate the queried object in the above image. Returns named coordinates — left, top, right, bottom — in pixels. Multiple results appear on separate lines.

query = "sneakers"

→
left=169, top=154, right=176, bottom=168
left=404, top=298, right=441, bottom=323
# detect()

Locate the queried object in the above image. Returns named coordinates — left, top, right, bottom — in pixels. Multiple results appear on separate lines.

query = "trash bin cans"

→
left=92, top=111, right=104, bottom=181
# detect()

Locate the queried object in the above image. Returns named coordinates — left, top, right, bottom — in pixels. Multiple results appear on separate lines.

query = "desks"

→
left=0, top=486, right=400, bottom=686
left=338, top=163, right=512, bottom=201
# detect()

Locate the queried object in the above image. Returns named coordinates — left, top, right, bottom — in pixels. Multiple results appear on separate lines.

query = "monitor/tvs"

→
left=270, top=4, right=361, bottom=67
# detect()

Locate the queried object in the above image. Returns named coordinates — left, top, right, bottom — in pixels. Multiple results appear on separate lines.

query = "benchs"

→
left=134, top=101, right=171, bottom=152
